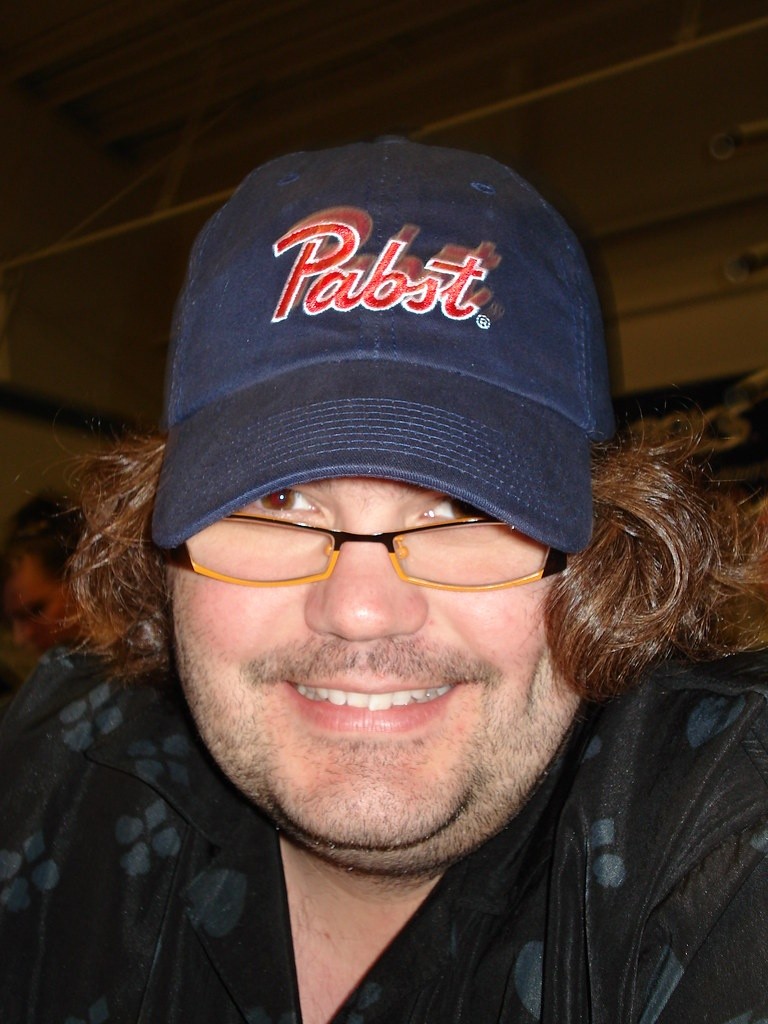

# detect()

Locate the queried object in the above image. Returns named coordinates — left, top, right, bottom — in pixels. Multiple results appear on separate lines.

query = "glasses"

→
left=144, top=510, right=569, bottom=592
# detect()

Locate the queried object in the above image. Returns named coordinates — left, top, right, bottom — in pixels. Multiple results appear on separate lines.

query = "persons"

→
left=0, top=139, right=768, bottom=1024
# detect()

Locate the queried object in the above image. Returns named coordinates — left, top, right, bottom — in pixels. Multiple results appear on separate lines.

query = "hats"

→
left=148, top=132, right=617, bottom=551
